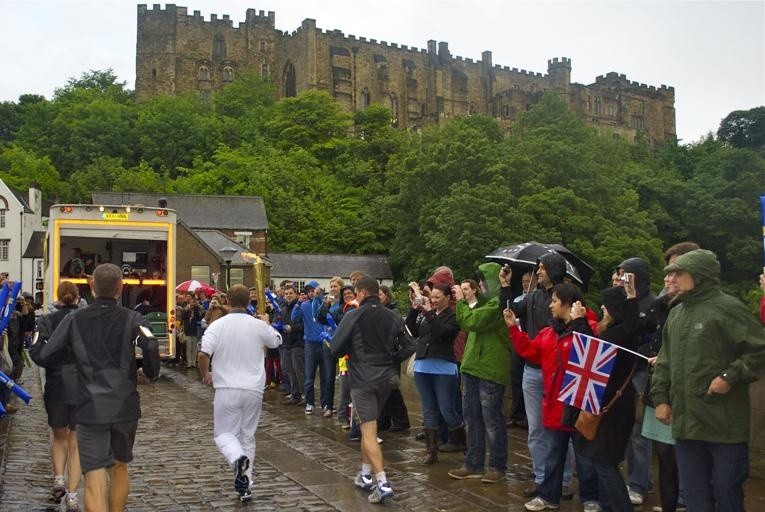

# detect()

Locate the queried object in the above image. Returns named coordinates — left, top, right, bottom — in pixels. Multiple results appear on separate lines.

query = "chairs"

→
left=64, top=493, right=79, bottom=511
left=523, top=484, right=652, bottom=511
left=354, top=470, right=373, bottom=489
left=233, top=455, right=249, bottom=492
left=368, top=481, right=395, bottom=504
left=240, top=489, right=253, bottom=501
left=47, top=477, right=66, bottom=504
left=304, top=403, right=351, bottom=428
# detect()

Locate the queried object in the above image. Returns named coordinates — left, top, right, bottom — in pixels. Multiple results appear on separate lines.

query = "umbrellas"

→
left=437, top=445, right=457, bottom=452
left=417, top=432, right=425, bottom=440
left=448, top=465, right=484, bottom=480
left=480, top=470, right=507, bottom=485
left=5, top=403, right=20, bottom=414
left=387, top=424, right=407, bottom=430
left=279, top=387, right=306, bottom=406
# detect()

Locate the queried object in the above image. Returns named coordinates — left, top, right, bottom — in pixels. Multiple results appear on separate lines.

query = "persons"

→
left=330, top=274, right=417, bottom=504
left=0, top=246, right=167, bottom=413
left=39, top=261, right=164, bottom=511
left=197, top=284, right=282, bottom=502
left=28, top=281, right=82, bottom=511
left=176, top=239, right=765, bottom=511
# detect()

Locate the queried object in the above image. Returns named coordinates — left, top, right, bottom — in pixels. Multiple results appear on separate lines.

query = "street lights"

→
left=574, top=409, right=604, bottom=439
left=406, top=353, right=415, bottom=380
left=640, top=407, right=676, bottom=445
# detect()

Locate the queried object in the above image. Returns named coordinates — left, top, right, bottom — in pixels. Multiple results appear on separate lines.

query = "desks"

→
left=218, top=246, right=238, bottom=288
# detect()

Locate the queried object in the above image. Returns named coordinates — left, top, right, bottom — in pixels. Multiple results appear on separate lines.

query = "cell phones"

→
left=621, top=273, right=629, bottom=282
left=415, top=297, right=424, bottom=305
left=327, top=295, right=335, bottom=302
left=503, top=265, right=511, bottom=274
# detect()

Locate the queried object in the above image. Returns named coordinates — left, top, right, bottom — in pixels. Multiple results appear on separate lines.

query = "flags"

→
left=557, top=333, right=618, bottom=416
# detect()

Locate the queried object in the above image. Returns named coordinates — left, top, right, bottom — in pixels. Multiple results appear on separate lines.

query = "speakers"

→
left=423, top=428, right=439, bottom=466
left=451, top=422, right=468, bottom=452
